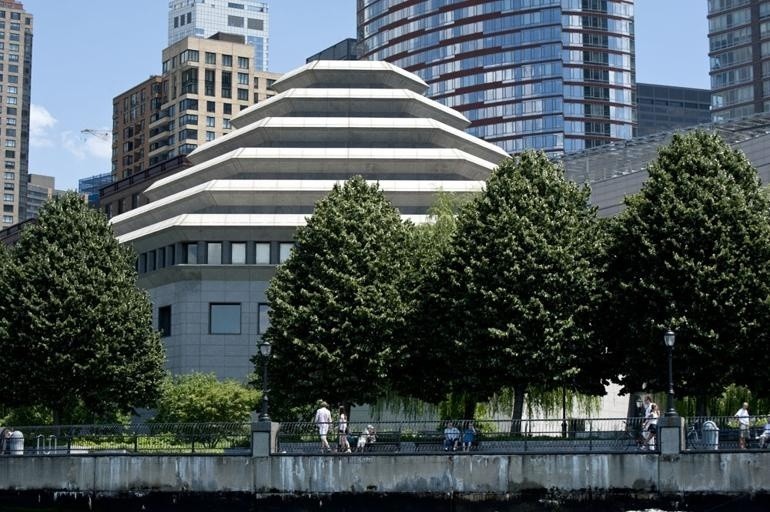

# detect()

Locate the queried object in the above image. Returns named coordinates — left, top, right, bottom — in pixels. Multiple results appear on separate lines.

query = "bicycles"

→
left=616, top=421, right=645, bottom=450
left=686, top=418, right=701, bottom=449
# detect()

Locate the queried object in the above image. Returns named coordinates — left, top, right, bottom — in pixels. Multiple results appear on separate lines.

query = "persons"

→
left=368, top=426, right=377, bottom=441
left=734, top=402, right=750, bottom=448
left=338, top=405, right=352, bottom=454
left=355, top=424, right=372, bottom=451
left=759, top=413, right=770, bottom=449
left=461, top=422, right=477, bottom=452
left=644, top=395, right=655, bottom=451
left=315, top=400, right=332, bottom=452
left=443, top=420, right=461, bottom=451
left=642, top=403, right=661, bottom=448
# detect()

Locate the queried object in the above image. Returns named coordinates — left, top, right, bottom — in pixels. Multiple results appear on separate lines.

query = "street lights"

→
left=258, top=341, right=272, bottom=421
left=636, top=399, right=643, bottom=416
left=664, top=327, right=678, bottom=416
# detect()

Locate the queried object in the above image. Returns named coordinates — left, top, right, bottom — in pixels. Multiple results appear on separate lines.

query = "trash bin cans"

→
left=9, top=431, right=24, bottom=455
left=701, top=421, right=720, bottom=450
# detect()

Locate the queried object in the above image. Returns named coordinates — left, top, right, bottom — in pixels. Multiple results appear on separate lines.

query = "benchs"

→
left=624, top=420, right=770, bottom=450
left=339, top=429, right=485, bottom=451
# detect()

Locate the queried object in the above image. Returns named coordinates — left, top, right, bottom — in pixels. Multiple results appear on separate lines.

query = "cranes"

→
left=80, top=127, right=112, bottom=142
